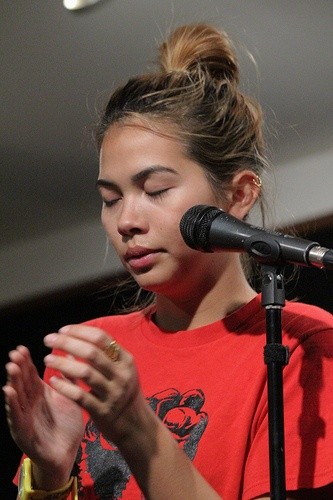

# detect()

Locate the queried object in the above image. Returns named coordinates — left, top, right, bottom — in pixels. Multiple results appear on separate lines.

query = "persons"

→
left=3, top=19, right=333, bottom=500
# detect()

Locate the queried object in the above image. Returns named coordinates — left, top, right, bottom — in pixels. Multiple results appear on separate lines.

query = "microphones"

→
left=180, top=204, right=332, bottom=273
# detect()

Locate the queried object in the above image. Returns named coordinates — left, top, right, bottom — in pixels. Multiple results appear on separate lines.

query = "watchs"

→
left=17, top=455, right=78, bottom=500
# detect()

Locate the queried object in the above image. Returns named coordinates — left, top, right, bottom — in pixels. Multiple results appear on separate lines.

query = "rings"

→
left=103, top=338, right=122, bottom=362
